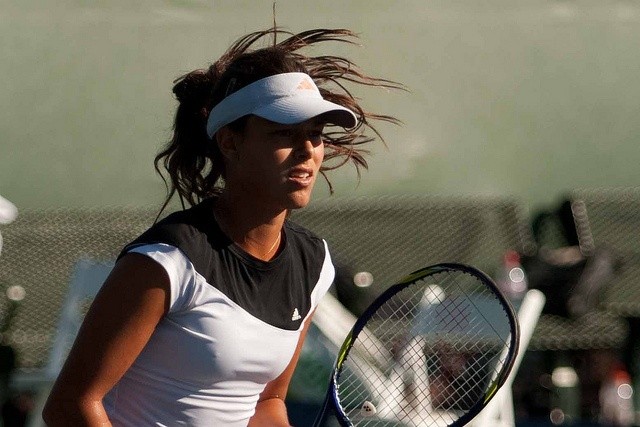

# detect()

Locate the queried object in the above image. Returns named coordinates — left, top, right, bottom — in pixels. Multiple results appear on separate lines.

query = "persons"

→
left=43, top=27, right=411, bottom=425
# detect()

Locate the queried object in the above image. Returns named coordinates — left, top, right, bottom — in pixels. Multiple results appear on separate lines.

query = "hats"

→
left=206, top=73, right=358, bottom=138
